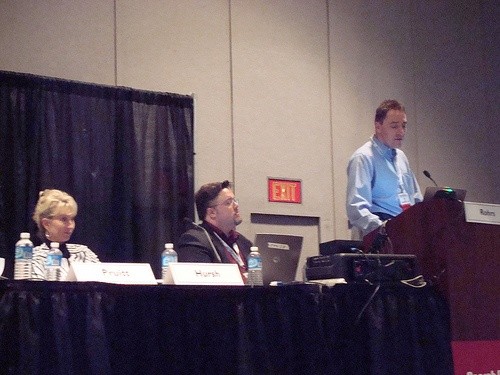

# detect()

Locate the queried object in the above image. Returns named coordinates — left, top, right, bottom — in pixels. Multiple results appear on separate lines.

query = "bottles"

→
left=14, top=232, right=34, bottom=280
left=161, top=242, right=178, bottom=285
left=247, top=246, right=264, bottom=286
left=44, top=241, right=63, bottom=281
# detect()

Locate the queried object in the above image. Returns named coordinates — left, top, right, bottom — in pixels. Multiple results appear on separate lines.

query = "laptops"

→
left=255, top=232, right=304, bottom=285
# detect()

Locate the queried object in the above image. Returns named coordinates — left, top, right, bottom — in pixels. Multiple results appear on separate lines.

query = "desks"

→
left=0, top=279, right=454, bottom=375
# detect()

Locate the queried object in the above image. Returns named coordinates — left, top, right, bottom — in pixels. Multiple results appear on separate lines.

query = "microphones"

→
left=183, top=216, right=221, bottom=264
left=422, top=170, right=438, bottom=187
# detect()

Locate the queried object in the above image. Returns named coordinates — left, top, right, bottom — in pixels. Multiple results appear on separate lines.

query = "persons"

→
left=174, top=180, right=255, bottom=284
left=346, top=99, right=424, bottom=241
left=3, top=189, right=78, bottom=279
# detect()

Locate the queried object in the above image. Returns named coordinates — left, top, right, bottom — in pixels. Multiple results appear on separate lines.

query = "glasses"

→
left=211, top=196, right=240, bottom=208
